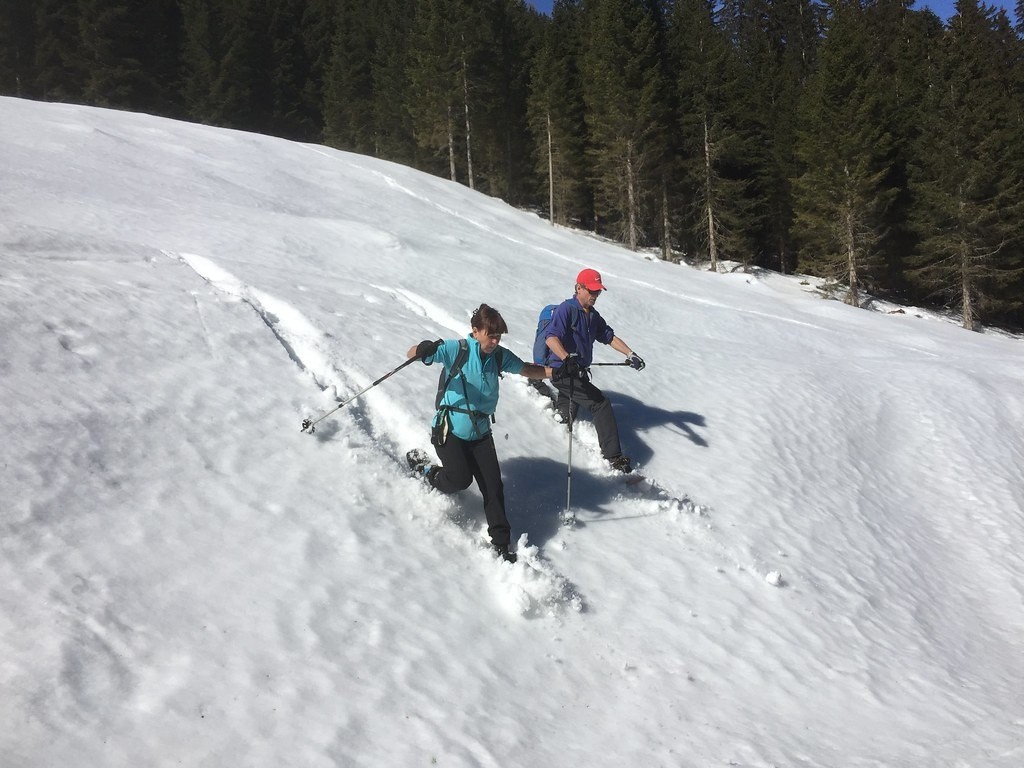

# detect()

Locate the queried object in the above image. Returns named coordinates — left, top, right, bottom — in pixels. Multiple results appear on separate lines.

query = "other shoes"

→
left=495, top=545, right=517, bottom=564
left=608, top=456, right=634, bottom=474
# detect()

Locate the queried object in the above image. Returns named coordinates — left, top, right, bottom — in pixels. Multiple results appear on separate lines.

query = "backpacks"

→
left=533, top=304, right=560, bottom=365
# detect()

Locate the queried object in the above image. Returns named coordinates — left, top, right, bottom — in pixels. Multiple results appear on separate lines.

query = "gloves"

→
left=628, top=352, right=646, bottom=372
left=416, top=339, right=438, bottom=358
left=562, top=357, right=588, bottom=373
left=552, top=362, right=579, bottom=382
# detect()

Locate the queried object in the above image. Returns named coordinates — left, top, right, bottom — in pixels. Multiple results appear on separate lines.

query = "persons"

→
left=544, top=269, right=646, bottom=476
left=407, top=304, right=582, bottom=564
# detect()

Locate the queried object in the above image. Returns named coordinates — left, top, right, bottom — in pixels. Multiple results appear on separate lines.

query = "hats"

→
left=576, top=269, right=607, bottom=291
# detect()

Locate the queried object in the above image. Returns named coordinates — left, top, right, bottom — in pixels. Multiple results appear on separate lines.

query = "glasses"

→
left=584, top=287, right=602, bottom=295
